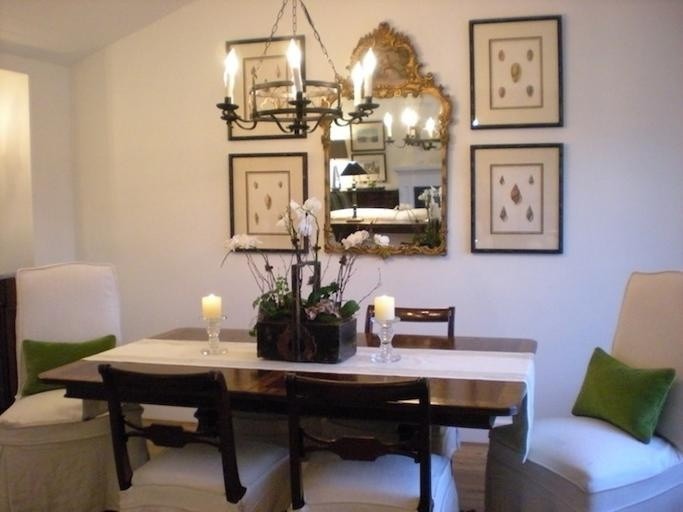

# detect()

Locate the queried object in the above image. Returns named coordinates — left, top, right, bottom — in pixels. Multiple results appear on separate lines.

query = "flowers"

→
left=226, top=198, right=392, bottom=323
left=403, top=182, right=442, bottom=245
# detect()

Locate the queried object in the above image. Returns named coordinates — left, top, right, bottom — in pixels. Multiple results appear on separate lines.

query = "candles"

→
left=372, top=294, right=395, bottom=319
left=200, top=294, right=223, bottom=319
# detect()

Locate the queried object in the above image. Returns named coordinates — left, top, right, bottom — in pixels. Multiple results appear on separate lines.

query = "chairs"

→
left=482, top=269, right=683, bottom=511
left=0, top=263, right=151, bottom=510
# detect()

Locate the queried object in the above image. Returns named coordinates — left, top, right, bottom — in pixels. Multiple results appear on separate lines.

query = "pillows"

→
left=19, top=333, right=119, bottom=398
left=570, top=346, right=675, bottom=447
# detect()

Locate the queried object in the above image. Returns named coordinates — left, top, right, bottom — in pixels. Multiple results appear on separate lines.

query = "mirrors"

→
left=317, top=22, right=450, bottom=259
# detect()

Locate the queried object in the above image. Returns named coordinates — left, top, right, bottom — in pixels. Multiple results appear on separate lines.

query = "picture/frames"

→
left=349, top=120, right=387, bottom=186
left=466, top=15, right=564, bottom=254
left=220, top=33, right=312, bottom=256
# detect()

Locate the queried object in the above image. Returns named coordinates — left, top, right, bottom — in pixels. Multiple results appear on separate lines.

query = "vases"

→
left=253, top=261, right=360, bottom=364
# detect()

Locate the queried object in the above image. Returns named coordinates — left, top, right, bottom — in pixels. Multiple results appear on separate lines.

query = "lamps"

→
left=382, top=106, right=441, bottom=152
left=215, top=1, right=379, bottom=136
left=327, top=139, right=349, bottom=191
left=340, top=161, right=369, bottom=223
